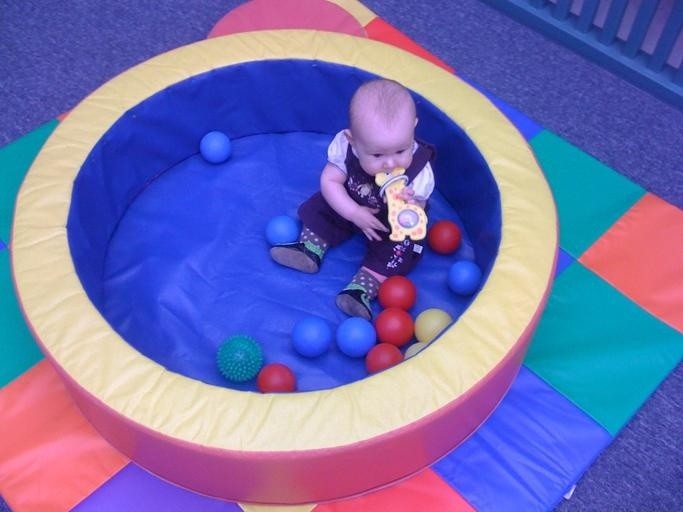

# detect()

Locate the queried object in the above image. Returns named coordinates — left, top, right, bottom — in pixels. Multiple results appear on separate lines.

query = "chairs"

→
left=336, top=288, right=373, bottom=321
left=269, top=242, right=321, bottom=273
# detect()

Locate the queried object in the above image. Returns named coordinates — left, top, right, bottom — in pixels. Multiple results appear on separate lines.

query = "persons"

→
left=269, top=78, right=436, bottom=321
